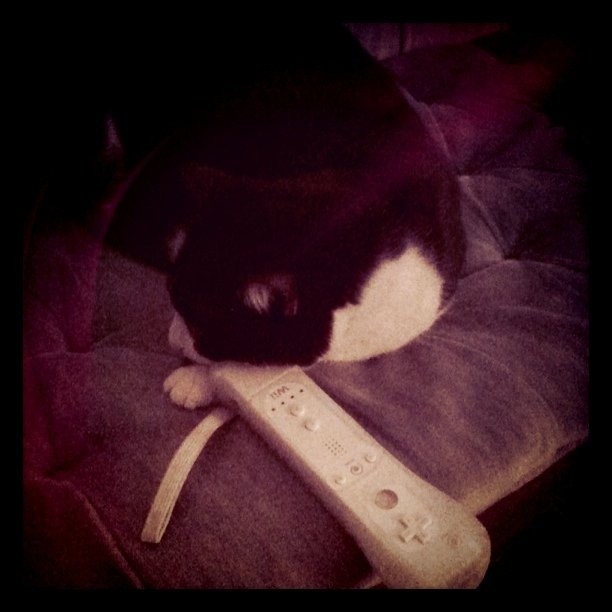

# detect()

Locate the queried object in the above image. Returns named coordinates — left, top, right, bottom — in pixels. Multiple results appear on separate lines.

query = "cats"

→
left=2, top=0, right=465, bottom=410
